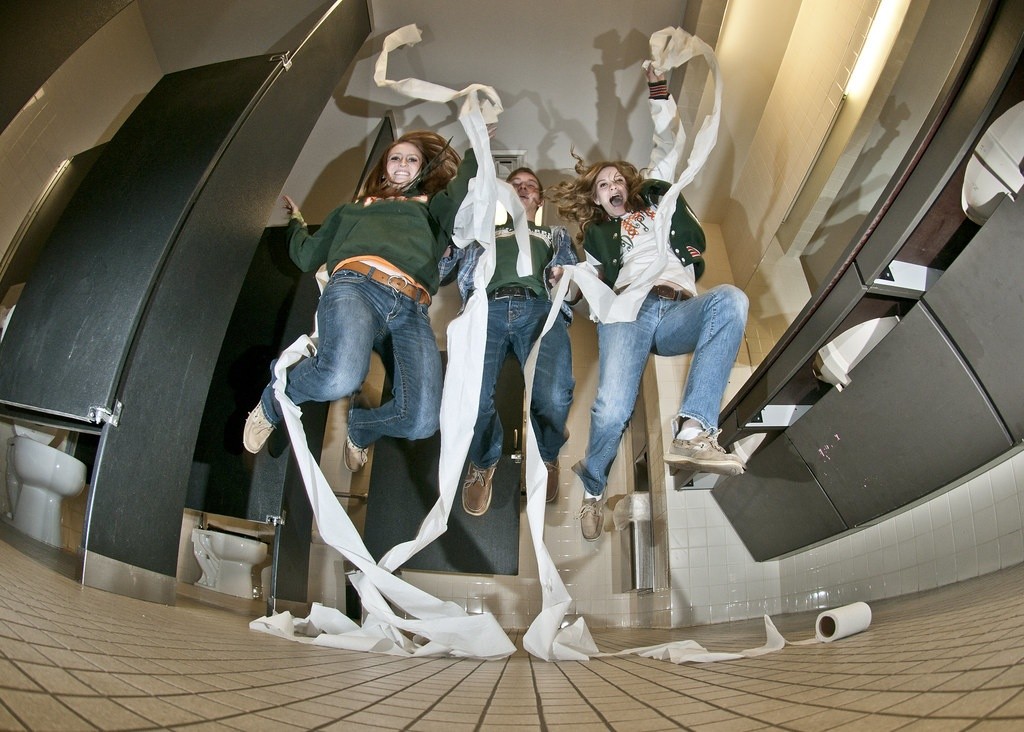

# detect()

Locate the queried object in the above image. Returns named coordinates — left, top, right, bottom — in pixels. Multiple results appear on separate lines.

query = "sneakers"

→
left=242, top=401, right=277, bottom=455
left=575, top=483, right=608, bottom=542
left=343, top=438, right=368, bottom=473
left=461, top=463, right=495, bottom=516
left=543, top=459, right=560, bottom=503
left=663, top=428, right=743, bottom=477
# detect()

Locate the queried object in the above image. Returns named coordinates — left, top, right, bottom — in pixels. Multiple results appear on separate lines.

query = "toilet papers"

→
left=815, top=601, right=872, bottom=643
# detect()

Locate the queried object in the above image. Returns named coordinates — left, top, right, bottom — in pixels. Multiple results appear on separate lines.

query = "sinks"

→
left=813, top=298, right=899, bottom=386
left=728, top=433, right=768, bottom=465
left=961, top=100, right=1024, bottom=226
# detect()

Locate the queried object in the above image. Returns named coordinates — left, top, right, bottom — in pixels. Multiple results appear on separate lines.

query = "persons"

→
left=436, top=167, right=579, bottom=516
left=243, top=120, right=495, bottom=475
left=537, top=62, right=749, bottom=542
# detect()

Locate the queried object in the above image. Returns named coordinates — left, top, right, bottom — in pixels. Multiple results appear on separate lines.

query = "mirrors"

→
left=744, top=0, right=982, bottom=373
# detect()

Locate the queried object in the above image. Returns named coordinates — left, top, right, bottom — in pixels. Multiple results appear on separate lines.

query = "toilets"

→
left=190, top=512, right=268, bottom=600
left=5, top=418, right=88, bottom=551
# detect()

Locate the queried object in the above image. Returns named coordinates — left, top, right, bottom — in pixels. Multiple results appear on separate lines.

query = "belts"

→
left=338, top=261, right=431, bottom=308
left=650, top=284, right=691, bottom=301
left=487, top=286, right=539, bottom=300
